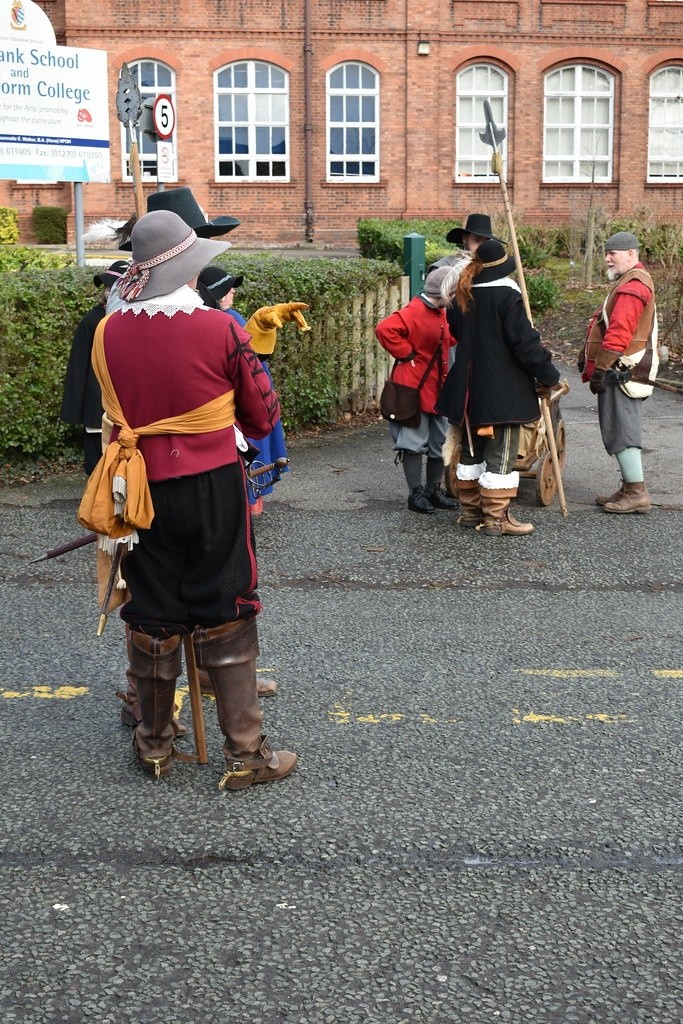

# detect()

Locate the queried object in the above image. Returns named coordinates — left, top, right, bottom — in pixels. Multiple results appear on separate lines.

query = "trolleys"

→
left=443, top=377, right=570, bottom=508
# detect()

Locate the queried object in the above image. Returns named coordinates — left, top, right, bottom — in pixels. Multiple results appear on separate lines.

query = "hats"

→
left=447, top=213, right=505, bottom=246
left=422, top=266, right=458, bottom=299
left=470, top=239, right=516, bottom=284
left=147, top=187, right=240, bottom=239
left=92, top=260, right=131, bottom=287
left=197, top=266, right=244, bottom=302
left=118, top=210, right=232, bottom=300
left=602, top=232, right=642, bottom=250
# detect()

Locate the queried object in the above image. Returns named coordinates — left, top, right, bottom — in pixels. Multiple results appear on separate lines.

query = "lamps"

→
left=418, top=41, right=430, bottom=56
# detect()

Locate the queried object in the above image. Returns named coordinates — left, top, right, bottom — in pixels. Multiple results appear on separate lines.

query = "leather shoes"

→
left=408, top=490, right=435, bottom=514
left=424, top=487, right=461, bottom=510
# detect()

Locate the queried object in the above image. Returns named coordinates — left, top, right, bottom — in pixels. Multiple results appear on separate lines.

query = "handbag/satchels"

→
left=616, top=345, right=659, bottom=398
left=380, top=379, right=420, bottom=429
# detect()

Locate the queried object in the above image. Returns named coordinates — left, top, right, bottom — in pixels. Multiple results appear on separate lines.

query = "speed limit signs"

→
left=153, top=94, right=176, bottom=139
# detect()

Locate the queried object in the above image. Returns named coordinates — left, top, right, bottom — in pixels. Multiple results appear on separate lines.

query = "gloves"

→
left=243, top=303, right=309, bottom=355
left=589, top=368, right=609, bottom=395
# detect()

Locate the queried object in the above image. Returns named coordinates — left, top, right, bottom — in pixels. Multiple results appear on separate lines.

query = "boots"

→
left=127, top=628, right=181, bottom=779
left=190, top=617, right=297, bottom=791
left=120, top=624, right=186, bottom=736
left=198, top=667, right=278, bottom=697
left=595, top=478, right=628, bottom=505
left=480, top=471, right=534, bottom=535
left=456, top=461, right=490, bottom=526
left=603, top=481, right=653, bottom=514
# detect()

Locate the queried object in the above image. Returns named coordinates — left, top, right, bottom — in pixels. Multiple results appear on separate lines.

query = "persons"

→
left=579, top=232, right=659, bottom=511
left=427, top=213, right=508, bottom=372
left=63, top=261, right=130, bottom=476
left=76, top=210, right=298, bottom=790
left=121, top=188, right=311, bottom=729
left=375, top=266, right=459, bottom=513
left=200, top=265, right=289, bottom=515
left=434, top=242, right=560, bottom=535
